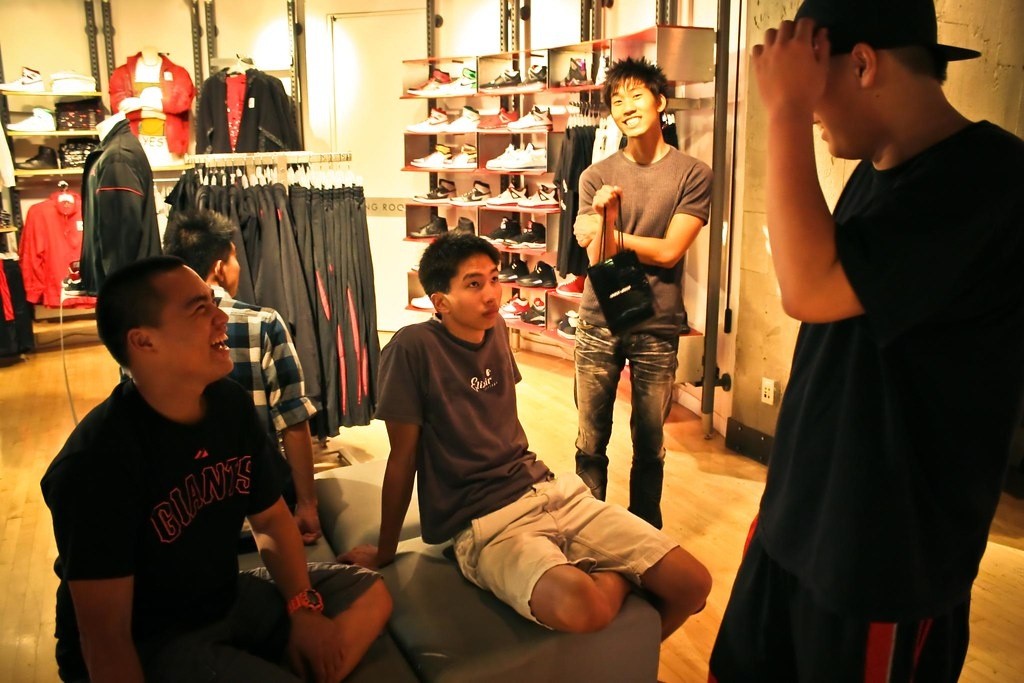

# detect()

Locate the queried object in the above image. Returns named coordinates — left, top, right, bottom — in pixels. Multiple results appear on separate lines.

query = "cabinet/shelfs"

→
left=0, top=90, right=103, bottom=178
left=399, top=24, right=714, bottom=384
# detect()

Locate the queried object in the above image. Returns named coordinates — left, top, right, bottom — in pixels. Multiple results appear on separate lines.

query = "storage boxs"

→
left=52, top=73, right=96, bottom=93
left=54, top=100, right=105, bottom=130
left=58, top=139, right=99, bottom=169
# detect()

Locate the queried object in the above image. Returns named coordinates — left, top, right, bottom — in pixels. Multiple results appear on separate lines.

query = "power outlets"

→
left=761, top=378, right=779, bottom=406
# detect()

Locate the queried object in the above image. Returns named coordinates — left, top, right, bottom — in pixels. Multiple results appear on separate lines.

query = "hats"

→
left=794, top=0, right=981, bottom=61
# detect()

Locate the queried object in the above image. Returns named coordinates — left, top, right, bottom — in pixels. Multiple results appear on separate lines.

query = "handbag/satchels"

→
left=589, top=195, right=659, bottom=336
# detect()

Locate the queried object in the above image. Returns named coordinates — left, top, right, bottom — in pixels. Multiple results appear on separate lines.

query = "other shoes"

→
left=62, top=261, right=87, bottom=296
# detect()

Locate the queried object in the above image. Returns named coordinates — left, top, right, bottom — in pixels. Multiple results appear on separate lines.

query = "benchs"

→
left=236, top=459, right=662, bottom=683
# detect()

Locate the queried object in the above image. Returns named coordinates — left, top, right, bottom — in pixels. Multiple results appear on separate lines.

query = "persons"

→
left=708, top=0, right=1024, bottom=683
left=108, top=42, right=195, bottom=160
left=162, top=208, right=322, bottom=548
left=78, top=109, right=163, bottom=297
left=36, top=253, right=393, bottom=683
left=571, top=54, right=716, bottom=530
left=336, top=229, right=714, bottom=644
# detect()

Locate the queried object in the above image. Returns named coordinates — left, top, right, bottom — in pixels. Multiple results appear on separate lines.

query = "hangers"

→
left=184, top=151, right=363, bottom=190
left=567, top=101, right=600, bottom=128
left=663, top=112, right=675, bottom=124
left=58, top=181, right=75, bottom=204
left=226, top=58, right=258, bottom=77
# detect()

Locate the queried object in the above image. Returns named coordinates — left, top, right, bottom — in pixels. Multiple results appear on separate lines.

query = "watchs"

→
left=286, top=588, right=324, bottom=616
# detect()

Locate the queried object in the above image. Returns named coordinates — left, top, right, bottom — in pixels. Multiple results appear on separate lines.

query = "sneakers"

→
left=410, top=105, right=553, bottom=132
left=413, top=178, right=562, bottom=209
left=406, top=56, right=609, bottom=93
left=0, top=67, right=46, bottom=92
left=409, top=213, right=546, bottom=247
left=412, top=291, right=578, bottom=339
left=410, top=143, right=546, bottom=171
left=5, top=107, right=56, bottom=131
left=411, top=259, right=585, bottom=298
left=15, top=146, right=56, bottom=169
